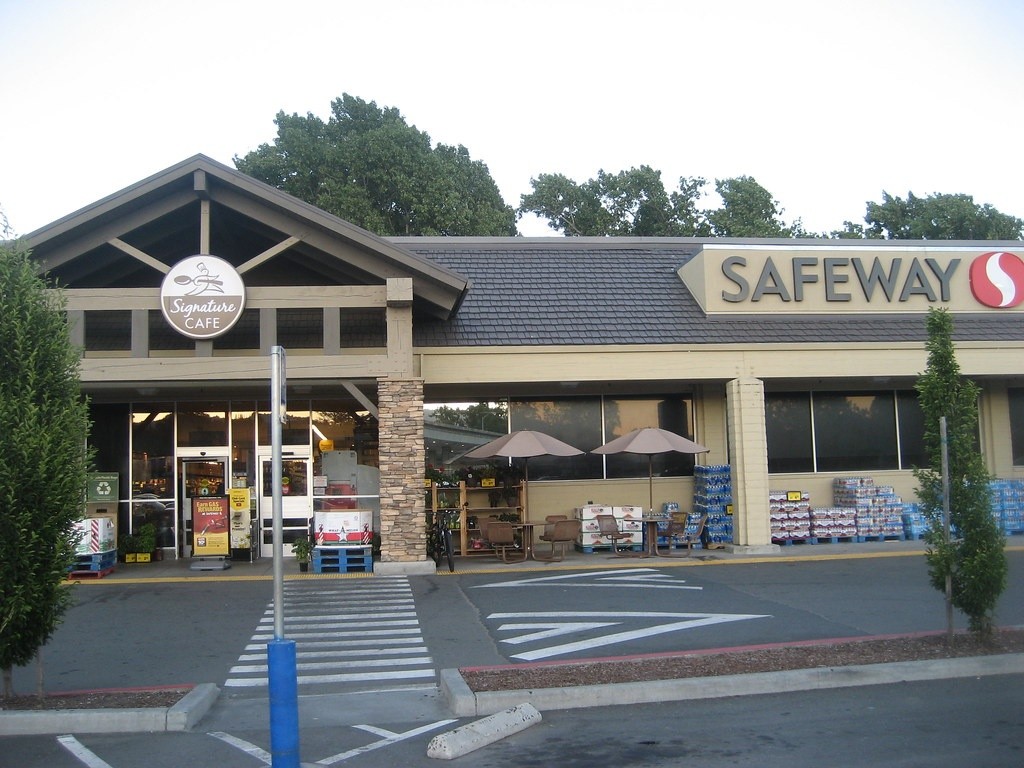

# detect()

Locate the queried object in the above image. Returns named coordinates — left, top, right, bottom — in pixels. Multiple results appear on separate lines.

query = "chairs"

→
left=660, top=514, right=709, bottom=558
left=477, top=517, right=514, bottom=564
left=539, top=515, right=582, bottom=563
left=657, top=512, right=688, bottom=554
left=596, top=515, right=634, bottom=557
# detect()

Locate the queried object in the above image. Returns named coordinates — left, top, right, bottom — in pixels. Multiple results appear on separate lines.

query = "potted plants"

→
left=291, top=538, right=310, bottom=572
left=136, top=524, right=157, bottom=563
left=466, top=515, right=478, bottom=529
left=426, top=463, right=523, bottom=487
left=118, top=534, right=136, bottom=563
left=488, top=492, right=501, bottom=507
left=503, top=485, right=518, bottom=506
left=438, top=492, right=460, bottom=528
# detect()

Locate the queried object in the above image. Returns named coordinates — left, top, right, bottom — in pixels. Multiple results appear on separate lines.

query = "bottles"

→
left=436, top=488, right=460, bottom=529
left=644, top=464, right=734, bottom=550
left=768, top=476, right=1024, bottom=543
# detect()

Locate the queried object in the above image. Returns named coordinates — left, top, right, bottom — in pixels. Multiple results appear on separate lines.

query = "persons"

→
left=133, top=484, right=166, bottom=537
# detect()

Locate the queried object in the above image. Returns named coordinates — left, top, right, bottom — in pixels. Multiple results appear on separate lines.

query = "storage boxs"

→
left=313, top=475, right=328, bottom=488
left=314, top=488, right=326, bottom=496
left=575, top=505, right=643, bottom=545
left=63, top=517, right=115, bottom=553
left=313, top=509, right=374, bottom=545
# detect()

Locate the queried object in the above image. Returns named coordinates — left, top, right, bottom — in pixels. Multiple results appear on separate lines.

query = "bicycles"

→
left=427, top=501, right=469, bottom=573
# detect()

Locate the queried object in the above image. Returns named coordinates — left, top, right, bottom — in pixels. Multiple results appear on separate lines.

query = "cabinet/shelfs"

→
left=426, top=485, right=524, bottom=552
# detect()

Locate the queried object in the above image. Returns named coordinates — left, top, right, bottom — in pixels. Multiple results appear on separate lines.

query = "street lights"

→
left=481, top=411, right=496, bottom=431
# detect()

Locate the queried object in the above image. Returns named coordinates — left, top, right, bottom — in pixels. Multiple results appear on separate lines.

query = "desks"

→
left=68, top=548, right=118, bottom=580
left=312, top=545, right=374, bottom=573
left=509, top=522, right=550, bottom=562
left=623, top=518, right=676, bottom=558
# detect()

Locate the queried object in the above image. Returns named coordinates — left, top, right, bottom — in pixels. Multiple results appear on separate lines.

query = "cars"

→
left=133, top=491, right=174, bottom=527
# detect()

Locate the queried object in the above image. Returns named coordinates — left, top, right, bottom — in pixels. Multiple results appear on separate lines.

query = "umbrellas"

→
left=464, top=430, right=587, bottom=547
left=590, top=427, right=710, bottom=519
left=442, top=440, right=502, bottom=467
